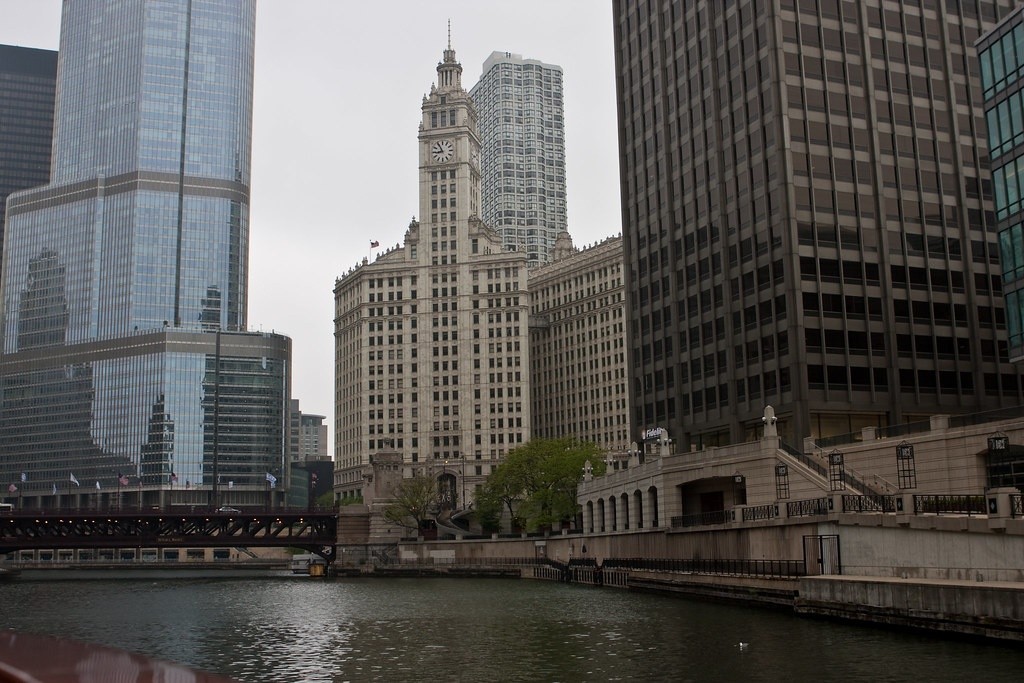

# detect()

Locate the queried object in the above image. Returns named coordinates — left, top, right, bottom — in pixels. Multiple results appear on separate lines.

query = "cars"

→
left=215, top=506, right=242, bottom=515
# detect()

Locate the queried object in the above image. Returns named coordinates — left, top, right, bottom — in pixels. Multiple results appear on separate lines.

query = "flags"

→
left=96, top=481, right=101, bottom=489
left=70, top=473, right=80, bottom=486
left=21, top=473, right=26, bottom=482
left=119, top=474, right=129, bottom=485
left=52, top=482, right=57, bottom=495
left=9, top=484, right=18, bottom=493
left=266, top=472, right=276, bottom=483
left=229, top=481, right=233, bottom=488
left=172, top=473, right=178, bottom=482
left=139, top=480, right=143, bottom=488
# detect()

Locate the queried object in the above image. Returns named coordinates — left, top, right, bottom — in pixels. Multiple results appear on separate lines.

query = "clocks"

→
left=431, top=139, right=454, bottom=164
